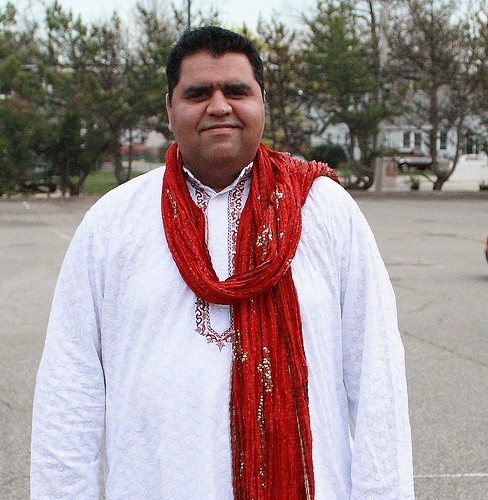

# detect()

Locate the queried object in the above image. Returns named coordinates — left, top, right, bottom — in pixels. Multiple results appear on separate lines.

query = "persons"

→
left=30, top=25, right=416, bottom=500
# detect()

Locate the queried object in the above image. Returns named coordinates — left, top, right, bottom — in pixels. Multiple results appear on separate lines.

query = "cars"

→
left=393, top=148, right=449, bottom=172
